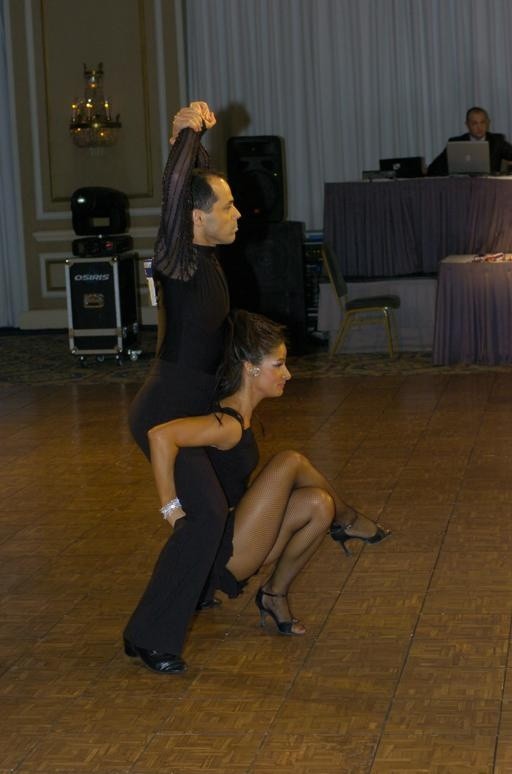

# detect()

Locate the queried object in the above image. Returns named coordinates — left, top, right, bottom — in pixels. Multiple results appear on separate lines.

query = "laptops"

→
left=445, top=141, right=491, bottom=174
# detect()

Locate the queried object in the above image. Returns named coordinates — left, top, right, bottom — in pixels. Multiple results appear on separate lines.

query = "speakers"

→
left=225, top=134, right=286, bottom=222
left=216, top=220, right=308, bottom=357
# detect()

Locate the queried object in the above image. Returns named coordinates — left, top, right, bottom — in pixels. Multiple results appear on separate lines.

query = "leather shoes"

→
left=123, top=637, right=189, bottom=673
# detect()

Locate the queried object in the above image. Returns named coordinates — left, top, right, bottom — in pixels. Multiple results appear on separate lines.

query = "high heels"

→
left=327, top=510, right=391, bottom=556
left=255, top=586, right=306, bottom=636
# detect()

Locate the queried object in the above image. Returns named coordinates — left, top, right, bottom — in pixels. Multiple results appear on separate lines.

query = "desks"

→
left=433, top=252, right=512, bottom=367
left=324, top=176, right=512, bottom=279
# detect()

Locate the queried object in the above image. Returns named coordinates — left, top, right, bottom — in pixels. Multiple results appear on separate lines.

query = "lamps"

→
left=69, top=62, right=122, bottom=148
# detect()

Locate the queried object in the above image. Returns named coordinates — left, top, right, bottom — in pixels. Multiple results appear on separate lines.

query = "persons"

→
left=147, top=309, right=392, bottom=638
left=426, top=106, right=512, bottom=178
left=123, top=102, right=242, bottom=675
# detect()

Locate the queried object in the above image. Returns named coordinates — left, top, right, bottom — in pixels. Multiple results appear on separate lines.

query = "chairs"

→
left=321, top=244, right=401, bottom=360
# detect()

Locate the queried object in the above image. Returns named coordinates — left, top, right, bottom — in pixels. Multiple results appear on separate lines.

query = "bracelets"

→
left=159, top=497, right=183, bottom=520
